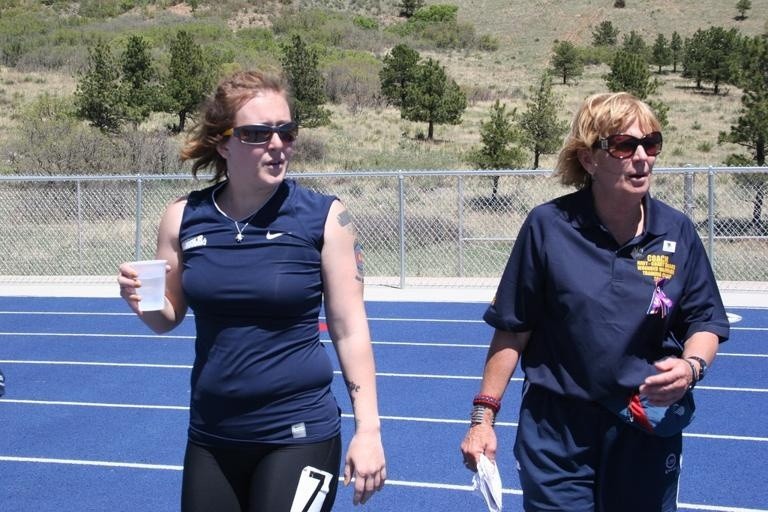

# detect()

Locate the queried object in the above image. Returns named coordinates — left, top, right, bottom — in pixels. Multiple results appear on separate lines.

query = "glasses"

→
left=593, top=129, right=665, bottom=162
left=220, top=120, right=302, bottom=148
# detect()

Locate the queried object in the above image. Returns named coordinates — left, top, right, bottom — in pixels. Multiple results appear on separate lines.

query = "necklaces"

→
left=223, top=186, right=277, bottom=243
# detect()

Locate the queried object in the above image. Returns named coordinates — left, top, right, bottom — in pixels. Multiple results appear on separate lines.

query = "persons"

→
left=460, top=88, right=733, bottom=512
left=116, top=70, right=389, bottom=512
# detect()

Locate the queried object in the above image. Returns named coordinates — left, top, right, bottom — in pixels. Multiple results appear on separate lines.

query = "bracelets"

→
left=471, top=393, right=502, bottom=411
left=682, top=356, right=698, bottom=393
left=469, top=407, right=495, bottom=427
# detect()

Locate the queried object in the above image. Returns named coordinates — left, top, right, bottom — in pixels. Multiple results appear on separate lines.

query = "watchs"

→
left=687, top=354, right=708, bottom=382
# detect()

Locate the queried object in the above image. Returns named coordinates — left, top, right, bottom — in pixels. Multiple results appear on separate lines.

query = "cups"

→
left=131, top=259, right=169, bottom=312
